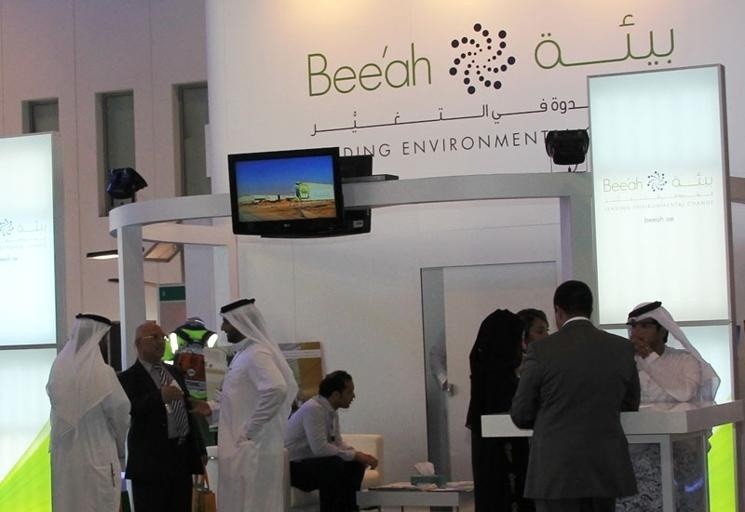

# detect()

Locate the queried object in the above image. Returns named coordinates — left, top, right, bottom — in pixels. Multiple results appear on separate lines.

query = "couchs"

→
left=206, top=433, right=384, bottom=512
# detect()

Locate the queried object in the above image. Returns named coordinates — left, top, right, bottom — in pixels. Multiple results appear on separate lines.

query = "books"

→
left=366, top=481, right=474, bottom=494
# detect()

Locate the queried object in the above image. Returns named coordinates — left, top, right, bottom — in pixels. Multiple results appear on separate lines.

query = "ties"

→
left=153, top=364, right=190, bottom=437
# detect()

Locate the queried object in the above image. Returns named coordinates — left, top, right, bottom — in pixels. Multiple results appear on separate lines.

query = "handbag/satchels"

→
left=191, top=466, right=216, bottom=512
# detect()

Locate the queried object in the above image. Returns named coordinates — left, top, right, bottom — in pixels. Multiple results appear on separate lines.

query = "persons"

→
left=464, top=307, right=529, bottom=511
left=285, top=370, right=379, bottom=511
left=515, top=308, right=550, bottom=345
left=44, top=310, right=132, bottom=511
left=625, top=300, right=721, bottom=454
left=117, top=321, right=208, bottom=511
left=507, top=280, right=643, bottom=512
left=214, top=297, right=300, bottom=511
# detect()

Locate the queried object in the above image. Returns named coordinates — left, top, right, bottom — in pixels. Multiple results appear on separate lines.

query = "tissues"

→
left=411, top=462, right=447, bottom=491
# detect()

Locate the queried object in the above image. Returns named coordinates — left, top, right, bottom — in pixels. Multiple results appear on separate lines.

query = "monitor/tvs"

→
left=261, top=155, right=372, bottom=238
left=228, top=147, right=346, bottom=236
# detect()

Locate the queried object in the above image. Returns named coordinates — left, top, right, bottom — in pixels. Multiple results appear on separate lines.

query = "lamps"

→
left=546, top=129, right=589, bottom=171
left=106, top=167, right=148, bottom=207
